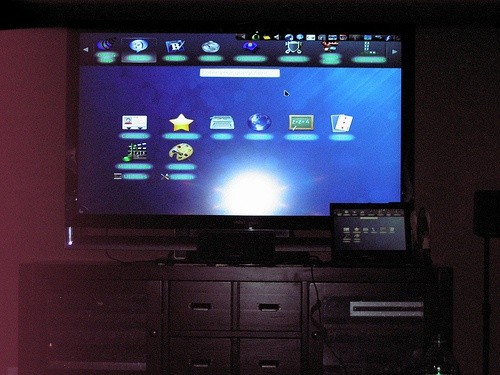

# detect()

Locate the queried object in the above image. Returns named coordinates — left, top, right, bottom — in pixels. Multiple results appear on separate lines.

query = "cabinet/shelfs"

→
left=18, top=255, right=455, bottom=375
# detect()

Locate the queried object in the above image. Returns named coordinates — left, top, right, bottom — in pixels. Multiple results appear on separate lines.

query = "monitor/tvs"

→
left=66, top=31, right=418, bottom=229
left=330, top=203, right=412, bottom=268
